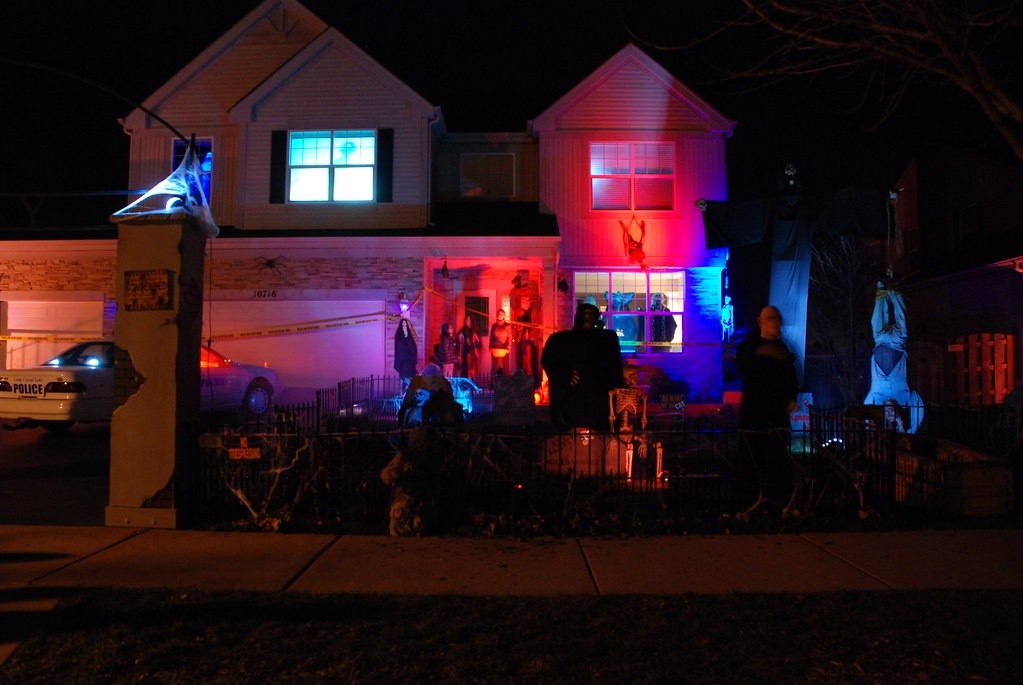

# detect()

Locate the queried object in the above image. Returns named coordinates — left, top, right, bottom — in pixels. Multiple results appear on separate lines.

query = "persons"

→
left=457, top=316, right=484, bottom=380
left=380, top=426, right=443, bottom=537
left=440, top=323, right=455, bottom=381
left=488, top=309, right=512, bottom=385
left=211, top=405, right=354, bottom=529
left=540, top=303, right=625, bottom=434
left=393, top=320, right=417, bottom=393
left=721, top=306, right=798, bottom=492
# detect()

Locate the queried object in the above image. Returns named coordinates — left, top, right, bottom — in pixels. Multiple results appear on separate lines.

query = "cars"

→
left=0, top=335, right=282, bottom=433
